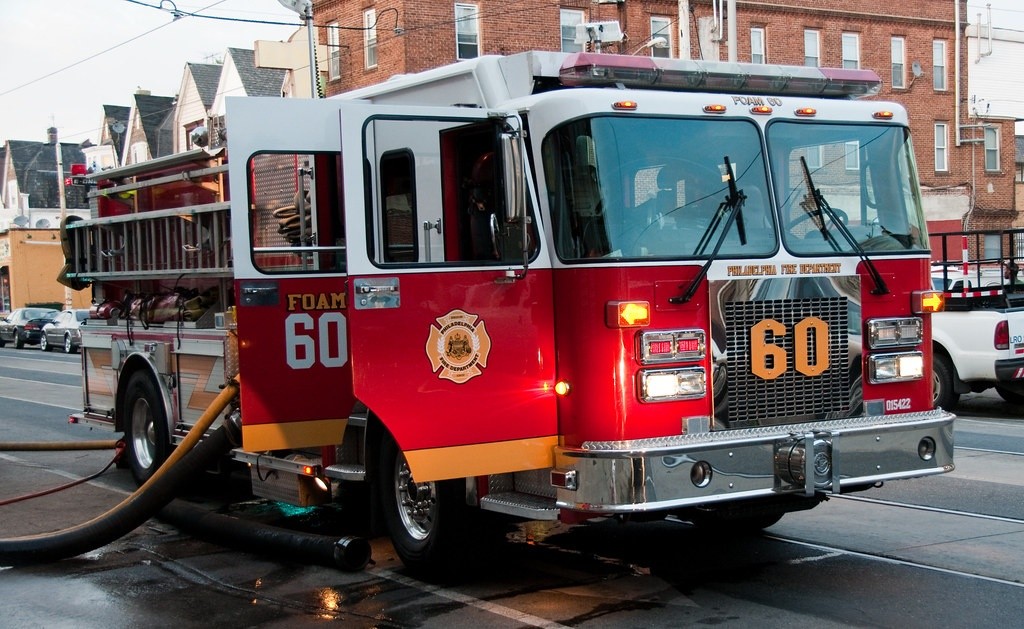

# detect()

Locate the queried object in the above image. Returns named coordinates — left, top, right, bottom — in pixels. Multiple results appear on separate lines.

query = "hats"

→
left=1004, top=263, right=1020, bottom=272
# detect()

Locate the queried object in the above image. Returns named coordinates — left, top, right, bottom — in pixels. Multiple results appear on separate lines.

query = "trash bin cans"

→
left=25, top=302, right=65, bottom=313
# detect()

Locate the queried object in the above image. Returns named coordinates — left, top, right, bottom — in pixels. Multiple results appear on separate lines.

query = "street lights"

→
left=280, top=0, right=317, bottom=98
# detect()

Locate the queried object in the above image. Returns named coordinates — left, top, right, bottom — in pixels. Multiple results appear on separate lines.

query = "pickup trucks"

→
left=931, top=304, right=1024, bottom=411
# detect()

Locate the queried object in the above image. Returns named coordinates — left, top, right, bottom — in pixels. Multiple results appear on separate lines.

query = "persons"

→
left=722, top=180, right=806, bottom=251
left=1003, top=262, right=1024, bottom=285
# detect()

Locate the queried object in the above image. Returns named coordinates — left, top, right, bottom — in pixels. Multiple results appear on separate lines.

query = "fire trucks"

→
left=59, top=47, right=958, bottom=574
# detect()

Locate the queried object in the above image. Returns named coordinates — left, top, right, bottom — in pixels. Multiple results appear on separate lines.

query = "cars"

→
left=40, top=308, right=90, bottom=354
left=0, top=307, right=59, bottom=350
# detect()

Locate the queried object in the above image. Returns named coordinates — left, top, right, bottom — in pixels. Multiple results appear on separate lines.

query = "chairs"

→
left=720, top=185, right=765, bottom=230
